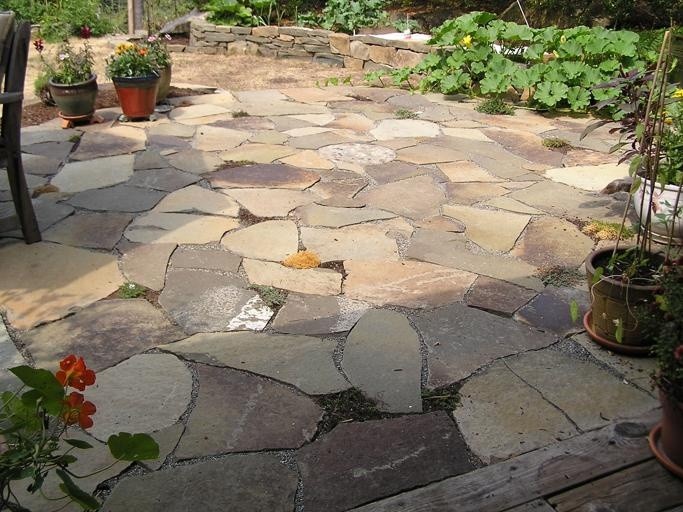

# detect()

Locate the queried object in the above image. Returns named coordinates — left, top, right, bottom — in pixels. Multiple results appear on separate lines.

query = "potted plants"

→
left=587, top=66, right=677, bottom=357
left=648, top=258, right=680, bottom=475
left=630, top=65, right=682, bottom=245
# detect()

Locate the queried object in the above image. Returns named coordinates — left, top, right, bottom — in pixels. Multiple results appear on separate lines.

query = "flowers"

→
left=34, top=22, right=96, bottom=83
left=104, top=40, right=162, bottom=78
left=135, top=32, right=173, bottom=65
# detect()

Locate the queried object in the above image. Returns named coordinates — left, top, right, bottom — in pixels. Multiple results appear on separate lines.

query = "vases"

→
left=146, top=62, right=172, bottom=103
left=111, top=75, right=158, bottom=118
left=49, top=73, right=99, bottom=117
